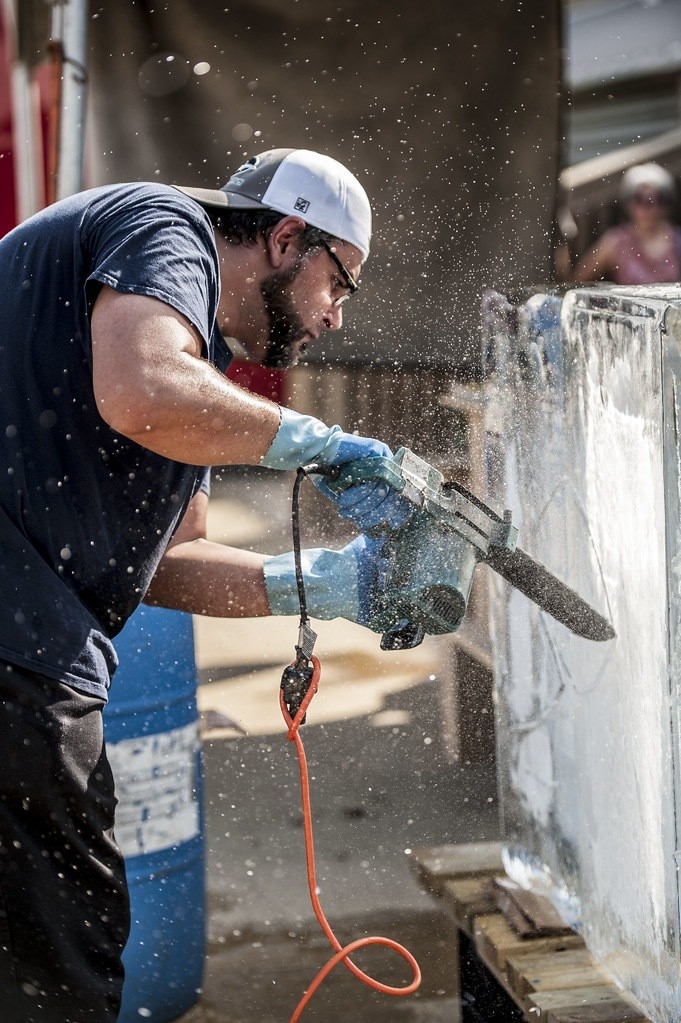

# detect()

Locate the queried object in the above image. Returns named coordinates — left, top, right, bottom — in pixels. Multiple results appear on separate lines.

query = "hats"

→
left=622, top=162, right=673, bottom=201
left=169, top=147, right=374, bottom=265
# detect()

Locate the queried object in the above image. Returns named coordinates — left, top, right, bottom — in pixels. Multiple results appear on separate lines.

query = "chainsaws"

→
left=323, top=442, right=616, bottom=651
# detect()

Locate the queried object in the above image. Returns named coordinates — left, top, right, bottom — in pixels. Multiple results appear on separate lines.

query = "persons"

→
left=0, top=146, right=411, bottom=1023
left=562, top=162, right=681, bottom=286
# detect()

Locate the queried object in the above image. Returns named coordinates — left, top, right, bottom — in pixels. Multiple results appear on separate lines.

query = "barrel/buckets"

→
left=103, top=600, right=207, bottom=1023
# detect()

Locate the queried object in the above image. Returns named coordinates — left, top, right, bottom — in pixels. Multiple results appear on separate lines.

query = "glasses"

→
left=309, top=228, right=361, bottom=309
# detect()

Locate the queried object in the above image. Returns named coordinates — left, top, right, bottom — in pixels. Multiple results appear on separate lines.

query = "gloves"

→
left=261, top=530, right=410, bottom=634
left=255, top=401, right=413, bottom=540
